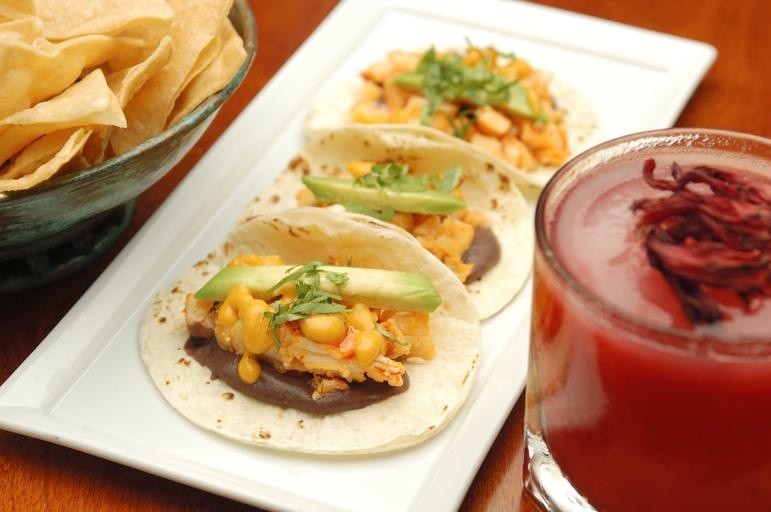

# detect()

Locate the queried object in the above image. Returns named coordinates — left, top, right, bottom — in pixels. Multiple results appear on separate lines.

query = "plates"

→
left=1, top=0, right=718, bottom=511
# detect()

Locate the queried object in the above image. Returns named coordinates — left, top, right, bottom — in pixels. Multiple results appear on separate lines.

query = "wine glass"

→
left=0, top=0, right=258, bottom=293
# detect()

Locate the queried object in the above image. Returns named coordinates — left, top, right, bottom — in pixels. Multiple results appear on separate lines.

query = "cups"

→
left=529, top=121, right=771, bottom=511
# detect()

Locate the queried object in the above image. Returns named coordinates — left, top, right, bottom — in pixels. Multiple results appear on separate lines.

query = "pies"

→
left=138, top=36, right=596, bottom=457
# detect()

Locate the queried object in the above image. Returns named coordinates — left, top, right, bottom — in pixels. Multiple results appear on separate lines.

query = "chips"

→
left=0, top=1, right=247, bottom=201
